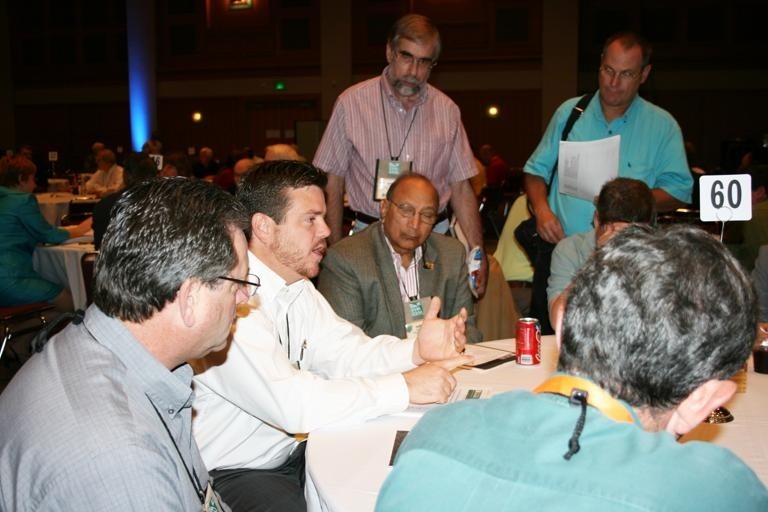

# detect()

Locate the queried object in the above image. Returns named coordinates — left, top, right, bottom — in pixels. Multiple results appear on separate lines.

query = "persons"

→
left=521, top=35, right=697, bottom=338
left=312, top=16, right=489, bottom=299
left=0, top=133, right=767, bottom=511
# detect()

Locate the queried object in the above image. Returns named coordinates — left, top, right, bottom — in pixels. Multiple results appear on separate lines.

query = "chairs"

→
left=472, top=252, right=528, bottom=341
left=29, top=311, right=85, bottom=353
left=60, top=212, right=93, bottom=226
left=69, top=199, right=99, bottom=213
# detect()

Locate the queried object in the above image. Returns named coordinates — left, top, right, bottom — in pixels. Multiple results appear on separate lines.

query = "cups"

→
left=752, top=345, right=767, bottom=374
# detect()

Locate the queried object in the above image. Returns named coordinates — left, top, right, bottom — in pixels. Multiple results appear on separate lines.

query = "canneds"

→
left=515, top=316, right=541, bottom=365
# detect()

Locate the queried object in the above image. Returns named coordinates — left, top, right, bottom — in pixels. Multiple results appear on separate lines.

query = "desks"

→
left=30, top=225, right=98, bottom=311
left=35, top=192, right=100, bottom=225
left=302, top=335, right=766, bottom=512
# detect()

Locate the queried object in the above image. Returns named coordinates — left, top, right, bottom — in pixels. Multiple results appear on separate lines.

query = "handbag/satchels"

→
left=513, top=213, right=542, bottom=271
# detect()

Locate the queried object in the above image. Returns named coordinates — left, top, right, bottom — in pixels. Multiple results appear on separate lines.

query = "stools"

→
left=0, top=301, right=54, bottom=367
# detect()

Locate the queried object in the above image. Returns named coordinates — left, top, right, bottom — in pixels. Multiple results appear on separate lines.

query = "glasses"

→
left=395, top=47, right=438, bottom=70
left=599, top=62, right=645, bottom=81
left=389, top=198, right=438, bottom=226
left=218, top=273, right=262, bottom=298
